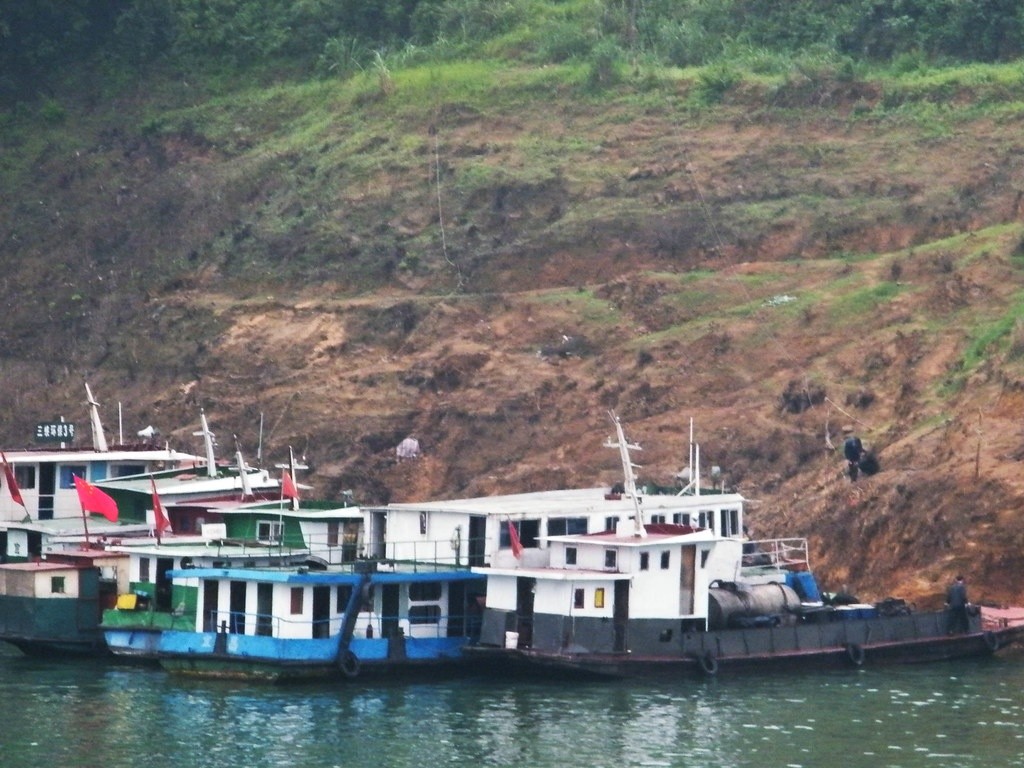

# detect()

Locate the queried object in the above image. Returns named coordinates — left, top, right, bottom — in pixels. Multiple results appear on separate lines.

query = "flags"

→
left=282, top=468, right=297, bottom=499
left=509, top=523, right=525, bottom=559
left=1, top=451, right=24, bottom=506
left=151, top=474, right=170, bottom=534
left=73, top=474, right=119, bottom=523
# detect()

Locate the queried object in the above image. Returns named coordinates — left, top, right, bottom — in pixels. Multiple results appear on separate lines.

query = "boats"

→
left=1, top=378, right=987, bottom=682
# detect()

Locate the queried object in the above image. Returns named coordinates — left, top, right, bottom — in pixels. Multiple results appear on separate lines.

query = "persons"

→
left=843, top=430, right=862, bottom=482
left=831, top=584, right=856, bottom=605
left=946, top=575, right=971, bottom=636
left=854, top=449, right=880, bottom=476
left=743, top=524, right=754, bottom=555
